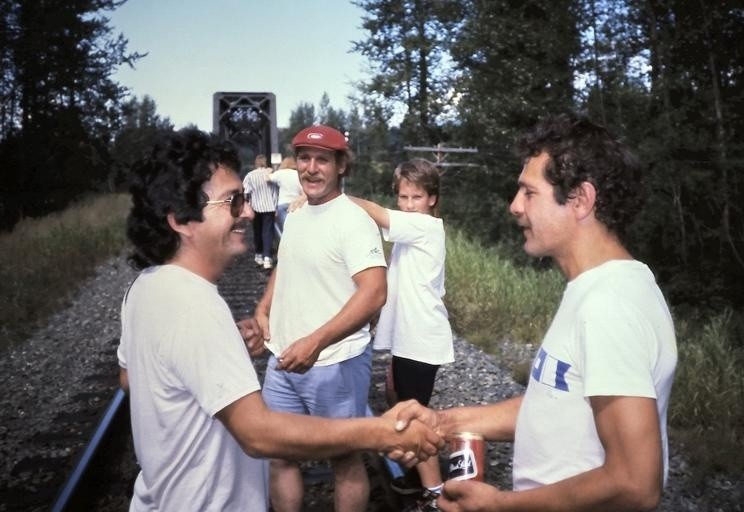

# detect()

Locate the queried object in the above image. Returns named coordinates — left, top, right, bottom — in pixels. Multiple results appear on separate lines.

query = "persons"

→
left=117, top=122, right=446, bottom=511
left=371, top=111, right=678, bottom=509
left=252, top=124, right=388, bottom=510
left=263, top=157, right=306, bottom=269
left=282, top=154, right=457, bottom=512
left=243, top=152, right=276, bottom=271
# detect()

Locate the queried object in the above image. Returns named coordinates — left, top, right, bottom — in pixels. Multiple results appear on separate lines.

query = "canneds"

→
left=449, top=433, right=484, bottom=481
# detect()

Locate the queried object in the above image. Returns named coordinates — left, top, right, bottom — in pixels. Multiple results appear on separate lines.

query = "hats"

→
left=291, top=125, right=349, bottom=152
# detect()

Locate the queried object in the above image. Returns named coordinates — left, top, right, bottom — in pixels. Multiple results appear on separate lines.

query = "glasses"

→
left=199, top=192, right=254, bottom=218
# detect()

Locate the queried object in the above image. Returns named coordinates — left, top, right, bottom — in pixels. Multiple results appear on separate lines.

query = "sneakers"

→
left=391, top=471, right=447, bottom=511
left=255, top=255, right=275, bottom=269
